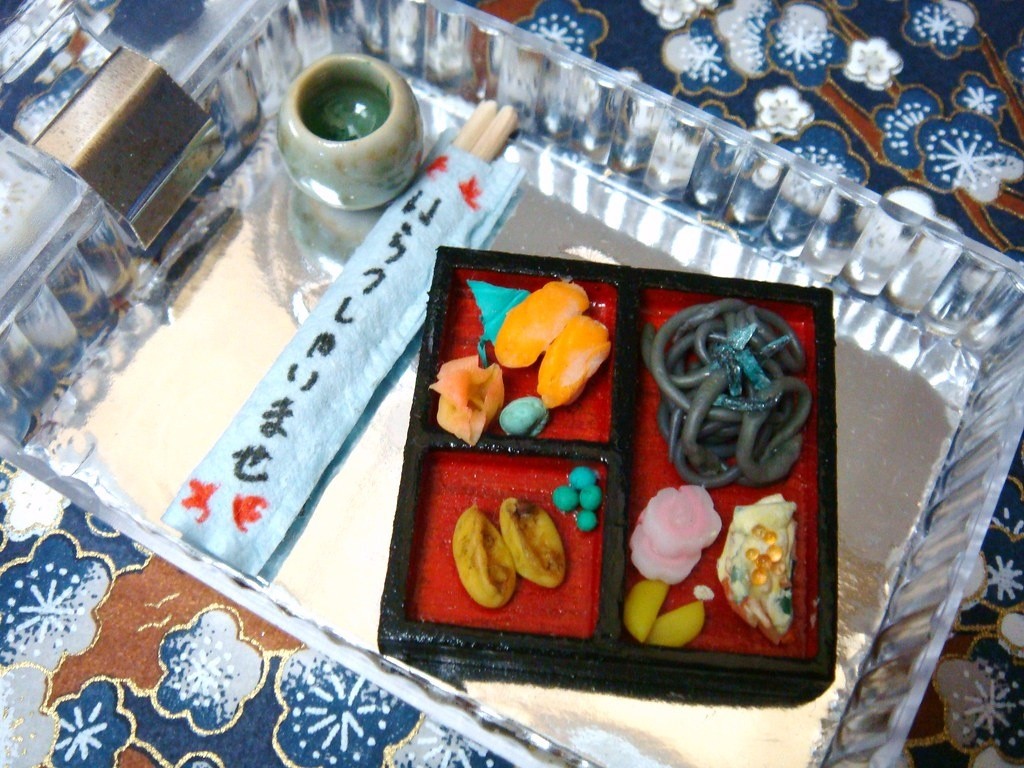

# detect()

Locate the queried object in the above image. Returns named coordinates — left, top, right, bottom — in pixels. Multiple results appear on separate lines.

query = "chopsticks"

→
left=452, top=96, right=519, bottom=163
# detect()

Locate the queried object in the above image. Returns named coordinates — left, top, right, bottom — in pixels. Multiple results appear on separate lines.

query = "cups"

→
left=276, top=52, right=423, bottom=211
